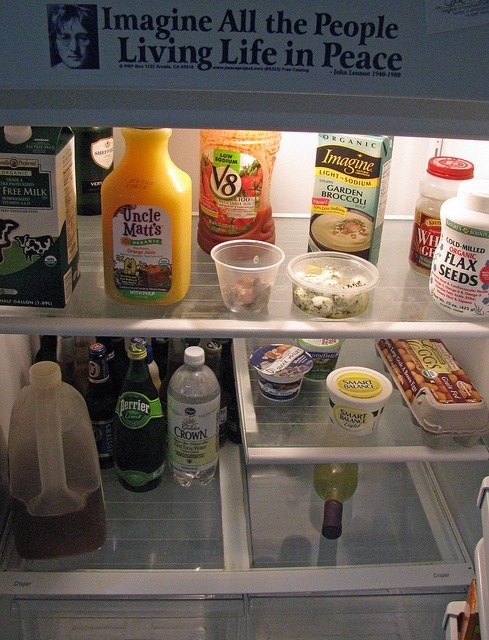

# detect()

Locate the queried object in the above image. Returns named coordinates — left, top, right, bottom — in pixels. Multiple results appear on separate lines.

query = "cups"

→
left=210, top=238, right=287, bottom=315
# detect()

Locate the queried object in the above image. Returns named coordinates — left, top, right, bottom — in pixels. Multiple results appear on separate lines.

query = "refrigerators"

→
left=0, top=1, right=489, bottom=640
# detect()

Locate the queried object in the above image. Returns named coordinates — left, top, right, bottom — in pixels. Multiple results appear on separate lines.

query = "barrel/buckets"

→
left=8, top=361, right=106, bottom=559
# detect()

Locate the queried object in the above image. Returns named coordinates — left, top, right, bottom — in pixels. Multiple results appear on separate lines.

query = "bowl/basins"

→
left=286, top=250, right=380, bottom=319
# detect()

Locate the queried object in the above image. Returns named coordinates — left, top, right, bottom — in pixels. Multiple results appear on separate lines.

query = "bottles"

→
left=112, top=344, right=166, bottom=493
left=211, top=338, right=231, bottom=356
left=73, top=335, right=92, bottom=394
left=111, top=337, right=128, bottom=371
left=179, top=338, right=214, bottom=371
left=129, top=336, right=147, bottom=346
left=100, top=127, right=192, bottom=307
left=151, top=337, right=168, bottom=382
left=197, top=129, right=282, bottom=262
left=206, top=387, right=227, bottom=448
left=98, top=342, right=124, bottom=395
left=428, top=179, right=489, bottom=318
left=312, top=462, right=360, bottom=541
left=144, top=344, right=163, bottom=396
left=158, top=336, right=187, bottom=453
left=84, top=342, right=117, bottom=471
left=71, top=126, right=114, bottom=216
left=203, top=341, right=233, bottom=382
left=55, top=335, right=81, bottom=394
left=165, top=346, right=221, bottom=490
left=223, top=399, right=242, bottom=444
